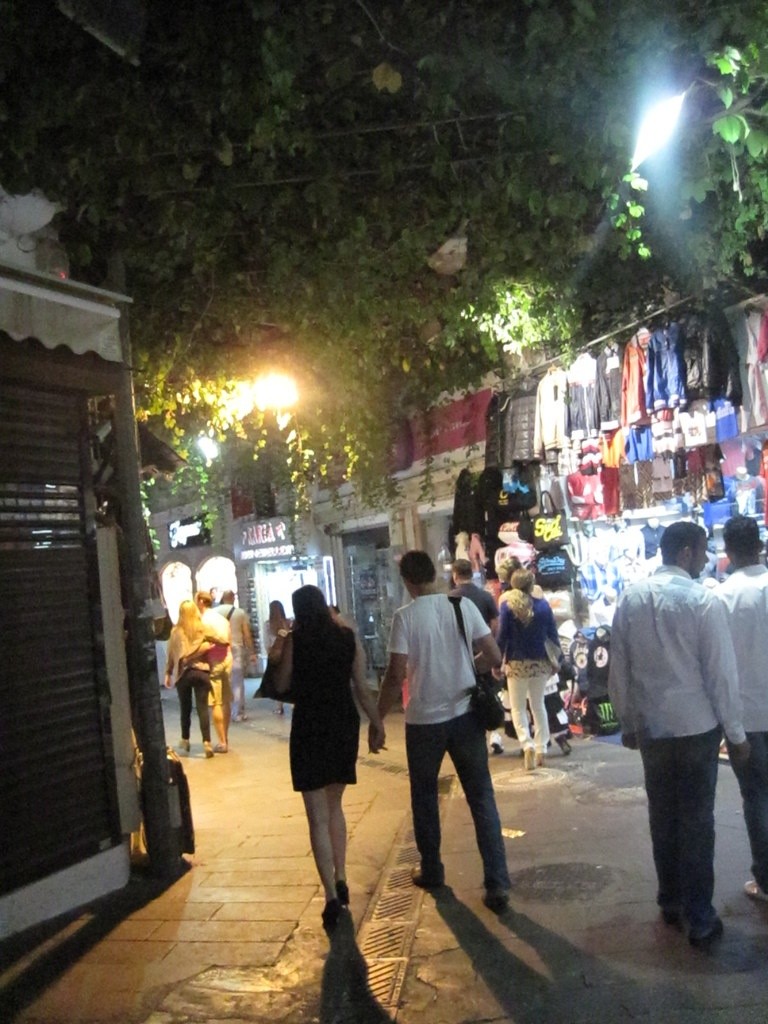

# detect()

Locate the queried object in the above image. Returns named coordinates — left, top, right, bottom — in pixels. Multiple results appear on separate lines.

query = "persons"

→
left=368, top=551, right=510, bottom=908
left=608, top=521, right=750, bottom=946
left=448, top=555, right=569, bottom=772
left=271, top=587, right=385, bottom=932
left=712, top=515, right=768, bottom=902
left=165, top=587, right=293, bottom=760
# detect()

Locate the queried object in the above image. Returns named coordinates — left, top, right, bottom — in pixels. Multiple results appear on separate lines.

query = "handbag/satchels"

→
left=535, top=548, right=572, bottom=586
left=470, top=680, right=506, bottom=731
left=533, top=489, right=570, bottom=550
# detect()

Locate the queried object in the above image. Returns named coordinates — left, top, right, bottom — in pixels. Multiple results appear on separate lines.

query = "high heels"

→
left=320, top=880, right=351, bottom=925
left=524, top=748, right=547, bottom=770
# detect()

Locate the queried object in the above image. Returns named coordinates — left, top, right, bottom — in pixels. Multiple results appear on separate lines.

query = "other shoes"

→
left=232, top=713, right=248, bottom=722
left=481, top=894, right=512, bottom=914
left=410, top=867, right=443, bottom=888
left=272, top=707, right=285, bottom=714
left=559, top=737, right=573, bottom=756
left=661, top=907, right=681, bottom=923
left=205, top=745, right=214, bottom=759
left=489, top=731, right=505, bottom=755
left=214, top=744, right=228, bottom=752
left=689, top=915, right=723, bottom=947
left=178, top=740, right=190, bottom=751
left=743, top=880, right=768, bottom=902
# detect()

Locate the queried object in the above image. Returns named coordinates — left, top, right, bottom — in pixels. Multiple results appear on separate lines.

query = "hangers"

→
left=523, top=299, right=705, bottom=384
